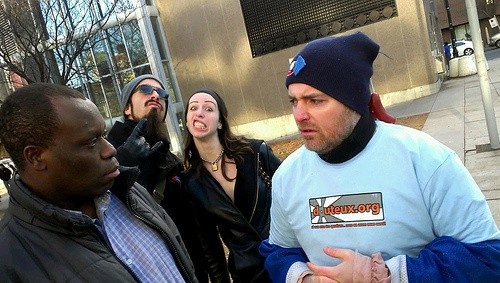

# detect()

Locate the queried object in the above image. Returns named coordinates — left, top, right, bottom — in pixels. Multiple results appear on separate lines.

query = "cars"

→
left=490, top=33, right=500, bottom=48
left=443, top=41, right=474, bottom=59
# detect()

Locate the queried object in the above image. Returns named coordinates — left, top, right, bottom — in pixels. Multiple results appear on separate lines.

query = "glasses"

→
left=131, top=84, right=169, bottom=101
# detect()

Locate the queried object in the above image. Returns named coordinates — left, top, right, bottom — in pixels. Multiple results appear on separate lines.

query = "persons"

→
left=0, top=82, right=199, bottom=283
left=106, top=74, right=210, bottom=283
left=444, top=41, right=451, bottom=70
left=184, top=88, right=282, bottom=283
left=0, top=164, right=12, bottom=190
left=259, top=31, right=500, bottom=283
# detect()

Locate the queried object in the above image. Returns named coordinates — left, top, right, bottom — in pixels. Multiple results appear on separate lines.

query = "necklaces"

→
left=201, top=146, right=224, bottom=171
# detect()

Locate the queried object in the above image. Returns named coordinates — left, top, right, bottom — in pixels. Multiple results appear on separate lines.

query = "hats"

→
left=121, top=74, right=168, bottom=120
left=286, top=31, right=380, bottom=115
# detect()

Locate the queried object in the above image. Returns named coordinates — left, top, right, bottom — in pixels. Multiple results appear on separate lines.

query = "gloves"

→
left=124, top=118, right=163, bottom=166
